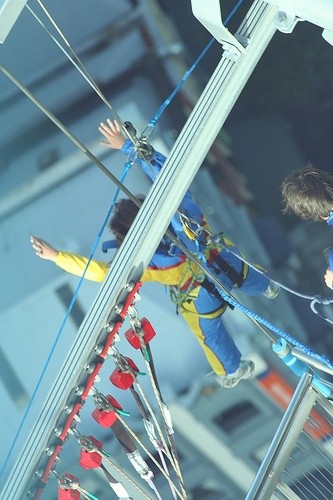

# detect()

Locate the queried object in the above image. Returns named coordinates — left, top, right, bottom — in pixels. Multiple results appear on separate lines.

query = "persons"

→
left=275, top=164, right=333, bottom=290
left=30, top=119, right=282, bottom=390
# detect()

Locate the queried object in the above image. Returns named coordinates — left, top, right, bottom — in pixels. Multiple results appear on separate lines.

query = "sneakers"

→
left=214, top=359, right=255, bottom=389
left=263, top=279, right=281, bottom=300
